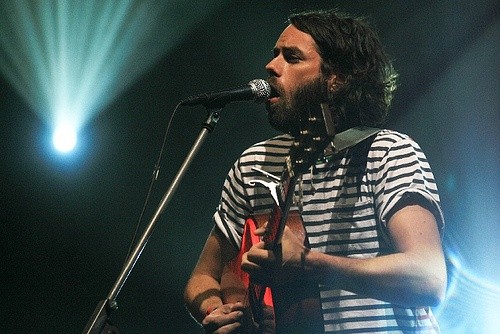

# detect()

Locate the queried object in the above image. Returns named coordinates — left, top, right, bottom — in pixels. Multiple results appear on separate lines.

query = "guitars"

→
left=220, top=102, right=336, bottom=334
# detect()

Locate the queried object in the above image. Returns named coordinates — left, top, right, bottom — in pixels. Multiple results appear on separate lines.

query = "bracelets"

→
left=206, top=306, right=219, bottom=314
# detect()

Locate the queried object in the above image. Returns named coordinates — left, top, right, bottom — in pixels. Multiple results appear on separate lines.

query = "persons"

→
left=184, top=10, right=447, bottom=334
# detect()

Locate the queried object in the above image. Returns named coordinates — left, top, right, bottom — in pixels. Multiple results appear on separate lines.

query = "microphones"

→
left=182, top=78, right=271, bottom=105
